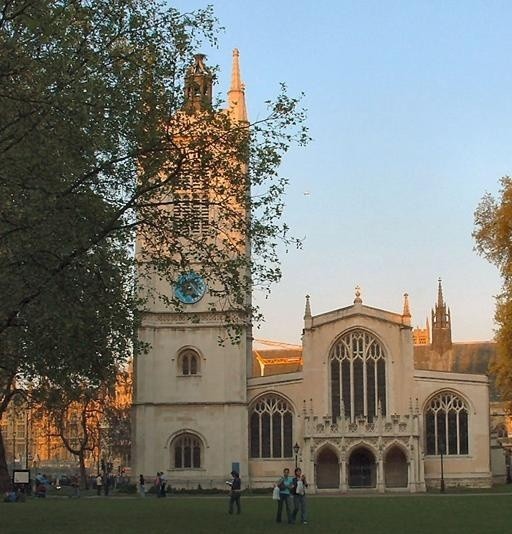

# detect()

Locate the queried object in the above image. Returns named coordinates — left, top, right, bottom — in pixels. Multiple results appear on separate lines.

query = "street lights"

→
left=10, top=431, right=16, bottom=472
left=437, top=439, right=447, bottom=493
left=293, top=442, right=300, bottom=469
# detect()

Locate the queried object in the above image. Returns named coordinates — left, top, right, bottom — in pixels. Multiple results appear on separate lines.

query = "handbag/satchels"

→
left=295, top=480, right=304, bottom=495
left=272, top=484, right=281, bottom=500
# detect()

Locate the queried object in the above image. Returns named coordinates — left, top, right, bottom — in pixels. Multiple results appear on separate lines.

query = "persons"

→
left=272, top=467, right=294, bottom=524
left=289, top=467, right=310, bottom=525
left=229, top=470, right=243, bottom=515
left=3, top=467, right=168, bottom=503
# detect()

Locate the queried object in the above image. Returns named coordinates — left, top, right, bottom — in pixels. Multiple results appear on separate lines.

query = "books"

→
left=225, top=479, right=233, bottom=486
